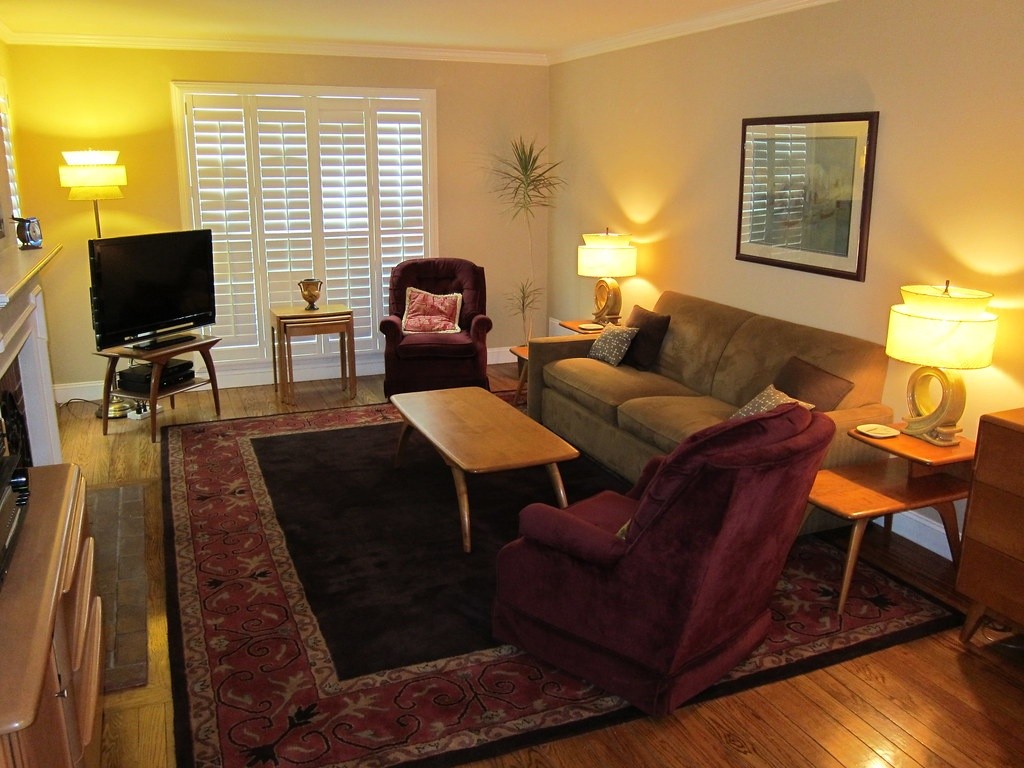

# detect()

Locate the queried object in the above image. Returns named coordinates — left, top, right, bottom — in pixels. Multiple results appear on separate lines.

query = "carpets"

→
left=159, top=389, right=966, bottom=767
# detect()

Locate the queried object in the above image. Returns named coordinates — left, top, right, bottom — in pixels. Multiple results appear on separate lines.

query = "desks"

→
left=270, top=305, right=357, bottom=406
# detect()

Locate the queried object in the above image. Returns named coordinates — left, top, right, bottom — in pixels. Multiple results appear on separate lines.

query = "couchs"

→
left=380, top=257, right=492, bottom=399
left=527, top=291, right=893, bottom=534
left=490, top=401, right=837, bottom=714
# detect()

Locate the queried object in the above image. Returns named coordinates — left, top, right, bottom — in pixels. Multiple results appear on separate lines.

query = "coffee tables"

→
left=390, top=387, right=580, bottom=553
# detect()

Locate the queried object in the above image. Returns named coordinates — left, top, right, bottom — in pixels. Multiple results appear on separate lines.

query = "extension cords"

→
left=127, top=405, right=163, bottom=419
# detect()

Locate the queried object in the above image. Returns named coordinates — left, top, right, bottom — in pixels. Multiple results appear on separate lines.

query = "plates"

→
left=579, top=324, right=603, bottom=329
left=857, top=423, right=901, bottom=439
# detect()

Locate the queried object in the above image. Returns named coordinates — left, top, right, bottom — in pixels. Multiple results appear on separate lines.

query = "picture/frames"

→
left=735, top=111, right=879, bottom=282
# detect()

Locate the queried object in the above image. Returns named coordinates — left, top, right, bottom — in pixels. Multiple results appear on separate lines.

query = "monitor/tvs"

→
left=88, top=230, right=216, bottom=350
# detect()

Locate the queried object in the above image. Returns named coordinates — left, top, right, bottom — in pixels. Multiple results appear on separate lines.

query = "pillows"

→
left=586, top=321, right=639, bottom=367
left=729, top=383, right=816, bottom=418
left=773, top=356, right=854, bottom=412
left=402, top=287, right=462, bottom=336
left=622, top=305, right=671, bottom=371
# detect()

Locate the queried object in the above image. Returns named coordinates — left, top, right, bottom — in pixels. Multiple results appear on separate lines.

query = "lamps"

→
left=578, top=227, right=637, bottom=326
left=885, top=280, right=999, bottom=447
left=59, top=147, right=138, bottom=419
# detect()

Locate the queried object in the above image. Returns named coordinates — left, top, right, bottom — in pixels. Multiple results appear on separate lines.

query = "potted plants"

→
left=489, top=132, right=568, bottom=379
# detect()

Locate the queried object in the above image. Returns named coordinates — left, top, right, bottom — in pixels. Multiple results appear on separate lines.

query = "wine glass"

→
left=299, top=278, right=322, bottom=310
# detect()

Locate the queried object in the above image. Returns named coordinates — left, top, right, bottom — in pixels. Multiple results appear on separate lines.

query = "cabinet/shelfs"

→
left=0, top=462, right=102, bottom=768
left=954, top=408, right=1024, bottom=643
left=797, top=422, right=975, bottom=615
left=93, top=332, right=221, bottom=444
left=510, top=320, right=605, bottom=403
